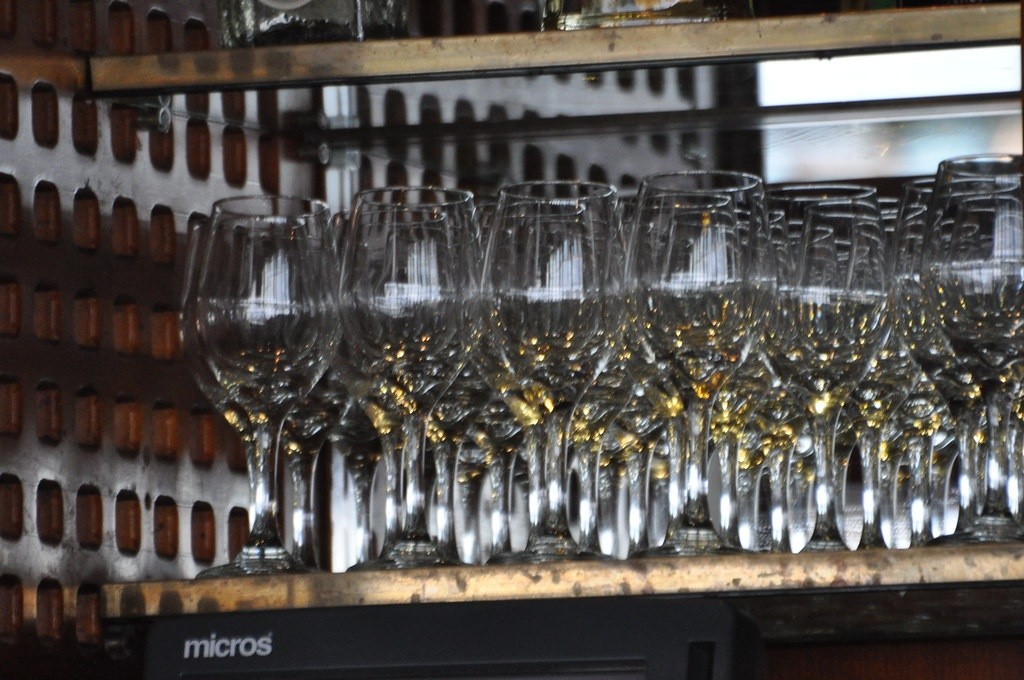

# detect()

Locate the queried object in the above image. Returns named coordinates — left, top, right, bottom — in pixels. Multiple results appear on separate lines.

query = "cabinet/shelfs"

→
left=0, top=0, right=1024, bottom=680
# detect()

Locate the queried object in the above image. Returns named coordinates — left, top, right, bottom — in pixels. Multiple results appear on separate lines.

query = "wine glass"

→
left=172, top=153, right=1024, bottom=577
